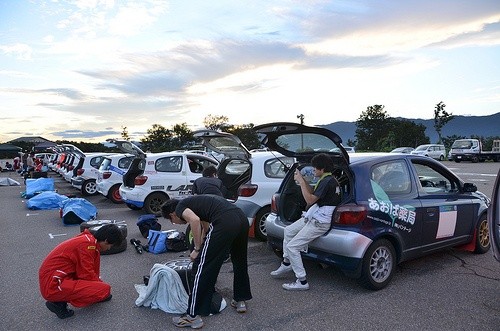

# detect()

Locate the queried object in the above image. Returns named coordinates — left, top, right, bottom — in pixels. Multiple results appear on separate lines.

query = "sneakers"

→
left=45, top=300, right=73, bottom=319
left=99, top=294, right=112, bottom=303
left=172, top=315, right=203, bottom=329
left=282, top=279, right=309, bottom=291
left=231, top=299, right=247, bottom=311
left=270, top=262, right=293, bottom=276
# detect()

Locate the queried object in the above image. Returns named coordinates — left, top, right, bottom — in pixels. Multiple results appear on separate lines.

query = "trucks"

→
left=450, top=138, right=500, bottom=162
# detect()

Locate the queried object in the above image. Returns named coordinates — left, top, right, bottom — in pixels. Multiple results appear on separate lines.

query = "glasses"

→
left=170, top=217, right=176, bottom=226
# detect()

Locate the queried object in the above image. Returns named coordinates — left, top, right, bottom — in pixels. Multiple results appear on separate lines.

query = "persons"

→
left=146, top=147, right=151, bottom=152
left=270, top=154, right=342, bottom=290
left=5, top=152, right=42, bottom=172
left=161, top=193, right=252, bottom=328
left=191, top=166, right=233, bottom=262
left=38, top=219, right=123, bottom=319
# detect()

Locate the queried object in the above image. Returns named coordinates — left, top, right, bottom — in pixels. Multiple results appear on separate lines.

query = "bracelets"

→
left=193, top=248, right=201, bottom=252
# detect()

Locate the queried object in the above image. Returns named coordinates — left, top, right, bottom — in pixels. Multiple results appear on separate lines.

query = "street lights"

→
left=297, top=114, right=305, bottom=151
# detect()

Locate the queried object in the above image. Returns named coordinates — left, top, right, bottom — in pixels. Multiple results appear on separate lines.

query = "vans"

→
left=329, top=146, right=355, bottom=154
left=410, top=144, right=446, bottom=161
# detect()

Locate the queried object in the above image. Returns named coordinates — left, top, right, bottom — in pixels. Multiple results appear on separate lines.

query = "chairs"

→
left=158, top=160, right=176, bottom=171
left=265, top=164, right=275, bottom=175
left=189, top=162, right=197, bottom=173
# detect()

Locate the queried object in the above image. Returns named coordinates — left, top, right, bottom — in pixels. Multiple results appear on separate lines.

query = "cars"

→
left=192, top=128, right=328, bottom=243
left=250, top=122, right=493, bottom=284
left=389, top=147, right=414, bottom=154
left=27, top=143, right=134, bottom=205
left=106, top=138, right=233, bottom=218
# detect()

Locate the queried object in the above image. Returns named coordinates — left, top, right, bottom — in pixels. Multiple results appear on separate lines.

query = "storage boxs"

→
left=31, top=171, right=49, bottom=179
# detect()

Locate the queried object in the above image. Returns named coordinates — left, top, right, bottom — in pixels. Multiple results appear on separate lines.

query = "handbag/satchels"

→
left=166, top=231, right=190, bottom=252
left=138, top=218, right=161, bottom=239
left=148, top=230, right=168, bottom=254
